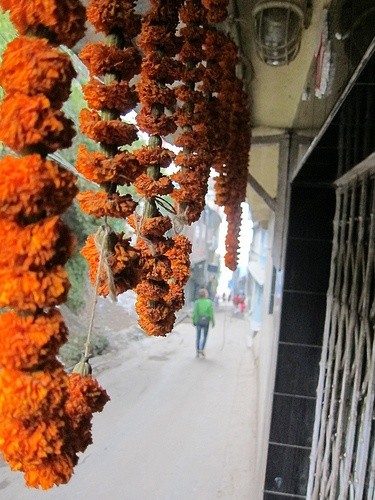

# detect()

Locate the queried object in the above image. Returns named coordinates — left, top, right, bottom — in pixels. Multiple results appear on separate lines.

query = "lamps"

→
left=249, top=0, right=307, bottom=67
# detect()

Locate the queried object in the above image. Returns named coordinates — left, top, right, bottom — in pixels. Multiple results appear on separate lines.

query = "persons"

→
left=192, top=288, right=216, bottom=356
left=221, top=291, right=246, bottom=315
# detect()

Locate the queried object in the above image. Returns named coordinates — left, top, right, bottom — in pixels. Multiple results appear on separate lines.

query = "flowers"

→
left=0, top=0, right=253, bottom=491
